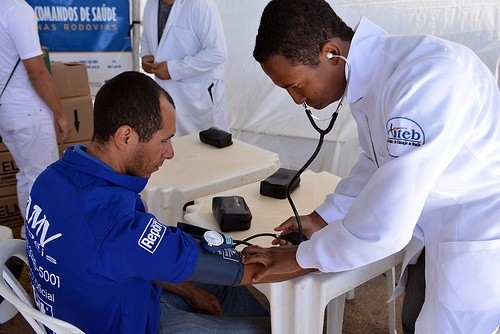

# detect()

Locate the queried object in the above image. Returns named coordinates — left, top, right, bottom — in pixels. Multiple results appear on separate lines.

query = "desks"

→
left=184, top=170, right=406, bottom=334
left=138, top=132, right=280, bottom=228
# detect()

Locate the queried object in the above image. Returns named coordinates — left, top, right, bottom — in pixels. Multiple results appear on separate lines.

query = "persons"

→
left=0, top=0, right=73, bottom=239
left=140, top=0, right=229, bottom=210
left=26, top=71, right=320, bottom=334
left=240, top=0, right=500, bottom=334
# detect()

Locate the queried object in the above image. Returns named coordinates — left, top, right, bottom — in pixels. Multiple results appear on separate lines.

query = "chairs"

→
left=0, top=238, right=86, bottom=334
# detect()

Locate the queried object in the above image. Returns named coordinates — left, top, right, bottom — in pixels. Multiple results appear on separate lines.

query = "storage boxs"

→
left=0, top=137, right=24, bottom=239
left=51, top=61, right=94, bottom=159
